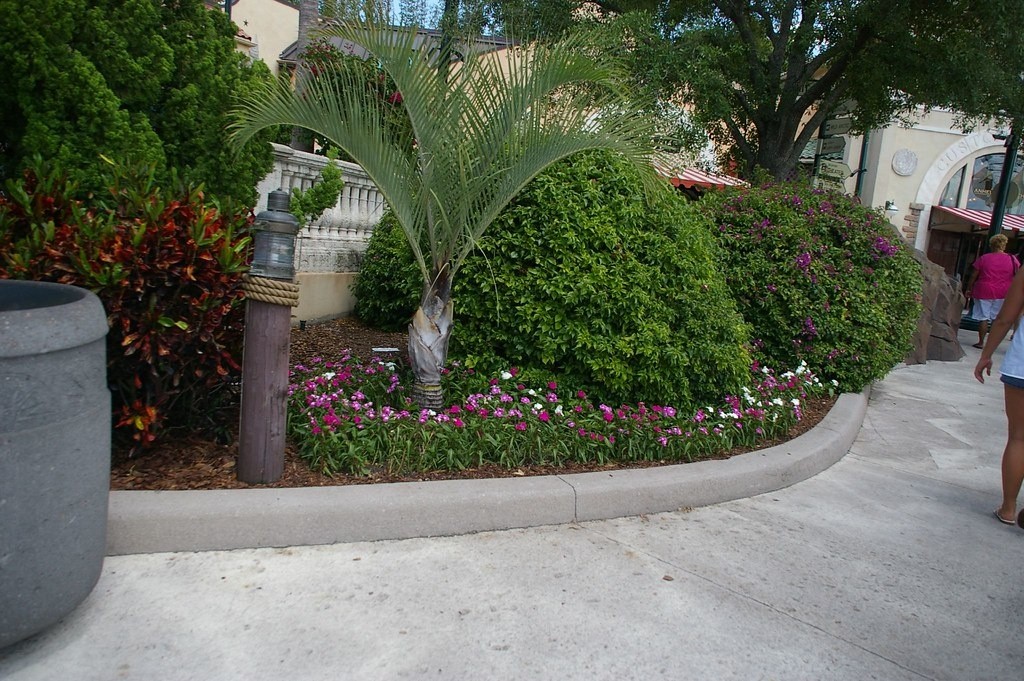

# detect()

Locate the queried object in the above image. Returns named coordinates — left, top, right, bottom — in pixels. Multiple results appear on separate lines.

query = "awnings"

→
left=648, top=158, right=752, bottom=189
left=928, top=206, right=1024, bottom=260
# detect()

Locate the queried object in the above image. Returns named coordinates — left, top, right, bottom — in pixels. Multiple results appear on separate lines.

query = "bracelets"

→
left=966, top=290, right=971, bottom=293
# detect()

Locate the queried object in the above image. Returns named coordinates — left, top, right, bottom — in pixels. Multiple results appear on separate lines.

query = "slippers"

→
left=993, top=507, right=1015, bottom=523
left=972, top=343, right=983, bottom=349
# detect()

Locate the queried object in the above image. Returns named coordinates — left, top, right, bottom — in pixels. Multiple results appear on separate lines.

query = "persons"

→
left=965, top=233, right=1021, bottom=349
left=974, top=266, right=1024, bottom=527
